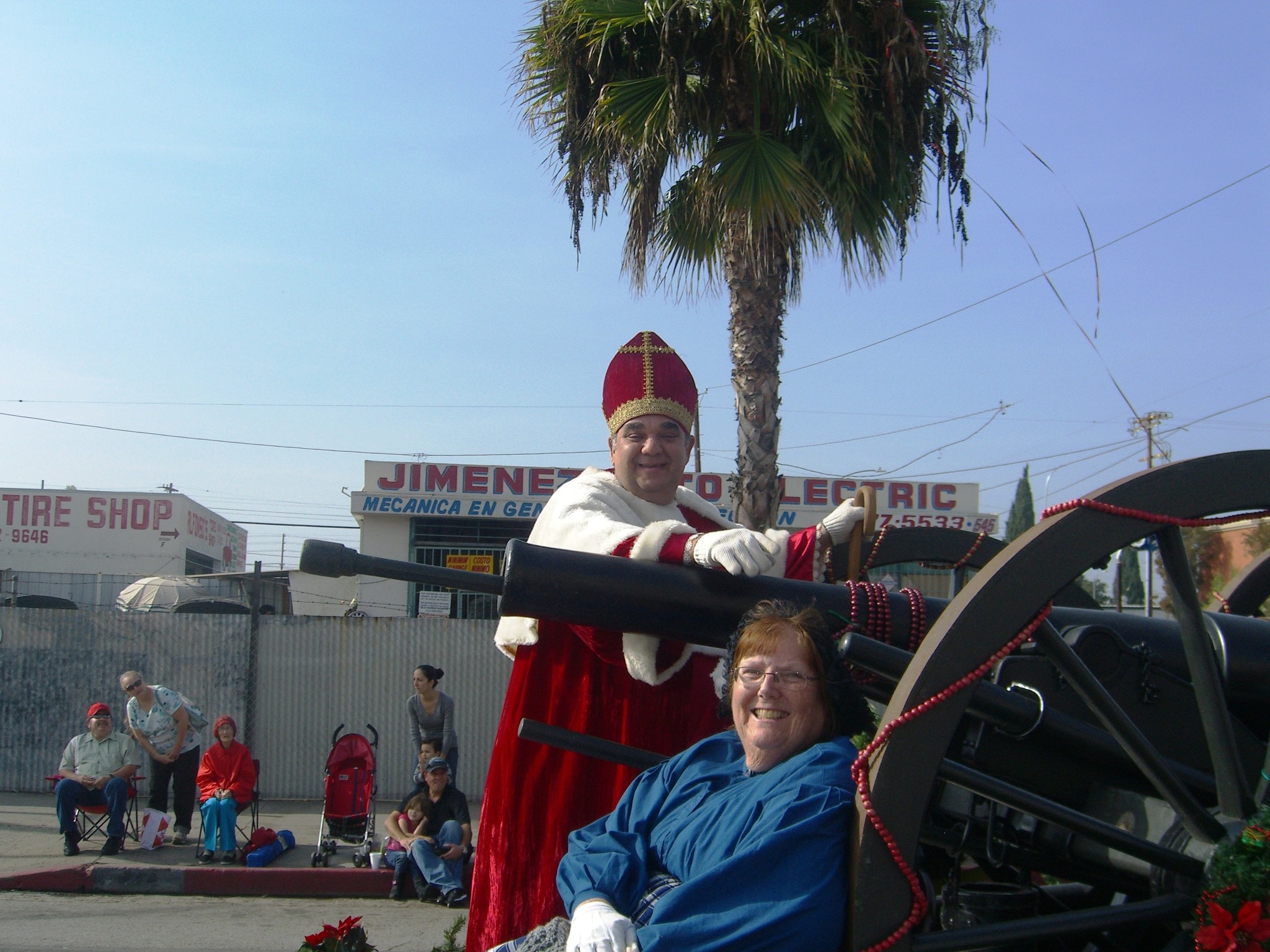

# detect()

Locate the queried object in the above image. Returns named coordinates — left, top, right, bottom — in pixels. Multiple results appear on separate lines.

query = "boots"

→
left=388, top=871, right=407, bottom=901
left=413, top=881, right=435, bottom=902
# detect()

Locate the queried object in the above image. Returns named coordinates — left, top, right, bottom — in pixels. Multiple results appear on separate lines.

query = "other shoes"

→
left=63, top=826, right=82, bottom=856
left=99, top=836, right=125, bottom=857
left=197, top=850, right=215, bottom=865
left=435, top=888, right=467, bottom=908
left=219, top=852, right=237, bottom=866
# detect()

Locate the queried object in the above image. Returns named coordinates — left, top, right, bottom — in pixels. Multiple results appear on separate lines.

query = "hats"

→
left=88, top=702, right=111, bottom=718
left=213, top=715, right=236, bottom=738
left=601, top=328, right=701, bottom=438
left=424, top=756, right=449, bottom=774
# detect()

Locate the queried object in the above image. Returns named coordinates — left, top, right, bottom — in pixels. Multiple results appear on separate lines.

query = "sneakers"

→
left=171, top=828, right=188, bottom=845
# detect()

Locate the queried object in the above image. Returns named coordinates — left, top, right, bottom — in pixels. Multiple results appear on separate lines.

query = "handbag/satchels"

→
left=154, top=685, right=210, bottom=733
left=138, top=807, right=171, bottom=851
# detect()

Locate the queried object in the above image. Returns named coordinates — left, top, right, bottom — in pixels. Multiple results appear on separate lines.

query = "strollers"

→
left=311, top=722, right=379, bottom=868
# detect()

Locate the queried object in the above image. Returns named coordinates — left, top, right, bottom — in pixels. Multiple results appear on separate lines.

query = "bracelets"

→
left=152, top=753, right=159, bottom=759
left=418, top=760, right=424, bottom=765
left=109, top=774, right=115, bottom=779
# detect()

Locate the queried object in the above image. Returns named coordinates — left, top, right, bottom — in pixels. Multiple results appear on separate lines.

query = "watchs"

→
left=461, top=844, right=468, bottom=854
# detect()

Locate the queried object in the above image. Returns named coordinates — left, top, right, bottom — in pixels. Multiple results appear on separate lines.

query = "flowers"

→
left=1195, top=900, right=1270, bottom=952
left=298, top=914, right=377, bottom=952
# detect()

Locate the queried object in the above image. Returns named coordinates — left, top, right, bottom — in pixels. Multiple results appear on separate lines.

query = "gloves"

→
left=563, top=901, right=638, bottom=952
left=694, top=526, right=780, bottom=579
left=821, top=496, right=868, bottom=547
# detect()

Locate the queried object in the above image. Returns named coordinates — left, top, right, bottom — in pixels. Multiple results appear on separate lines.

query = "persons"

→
left=382, top=665, right=473, bottom=908
left=467, top=332, right=865, bottom=952
left=54, top=702, right=141, bottom=856
left=120, top=671, right=208, bottom=844
left=196, top=716, right=257, bottom=864
left=484, top=599, right=876, bottom=952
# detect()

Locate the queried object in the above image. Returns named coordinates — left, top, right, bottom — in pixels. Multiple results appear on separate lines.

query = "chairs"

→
left=44, top=770, right=147, bottom=856
left=195, top=759, right=262, bottom=857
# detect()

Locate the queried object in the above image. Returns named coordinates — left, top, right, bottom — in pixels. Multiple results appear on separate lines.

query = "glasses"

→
left=731, top=666, right=822, bottom=690
left=123, top=677, right=142, bottom=692
left=88, top=712, right=114, bottom=720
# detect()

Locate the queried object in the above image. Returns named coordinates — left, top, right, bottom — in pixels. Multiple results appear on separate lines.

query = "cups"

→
left=369, top=852, right=382, bottom=870
